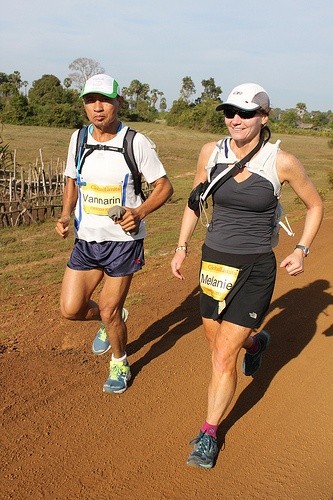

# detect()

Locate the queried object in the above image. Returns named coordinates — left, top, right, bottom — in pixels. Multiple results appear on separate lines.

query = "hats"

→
left=79, top=75, right=121, bottom=99
left=215, top=83, right=270, bottom=114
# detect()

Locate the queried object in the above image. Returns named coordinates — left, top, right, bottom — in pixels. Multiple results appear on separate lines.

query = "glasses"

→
left=223, top=109, right=263, bottom=119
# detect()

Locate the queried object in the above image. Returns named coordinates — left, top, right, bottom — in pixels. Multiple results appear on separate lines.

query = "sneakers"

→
left=240, top=331, right=270, bottom=376
left=187, top=431, right=219, bottom=468
left=102, top=361, right=131, bottom=394
left=91, top=308, right=128, bottom=355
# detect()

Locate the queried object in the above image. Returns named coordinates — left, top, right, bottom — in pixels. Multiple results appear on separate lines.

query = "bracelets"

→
left=175, top=247, right=188, bottom=251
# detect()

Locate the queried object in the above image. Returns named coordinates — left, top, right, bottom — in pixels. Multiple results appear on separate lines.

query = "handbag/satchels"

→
left=187, top=180, right=210, bottom=210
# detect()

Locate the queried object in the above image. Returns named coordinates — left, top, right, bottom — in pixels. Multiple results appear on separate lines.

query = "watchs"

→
left=295, top=245, right=310, bottom=257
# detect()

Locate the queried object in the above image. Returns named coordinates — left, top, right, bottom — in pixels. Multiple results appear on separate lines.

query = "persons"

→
left=55, top=75, right=174, bottom=393
left=171, top=84, right=324, bottom=469
